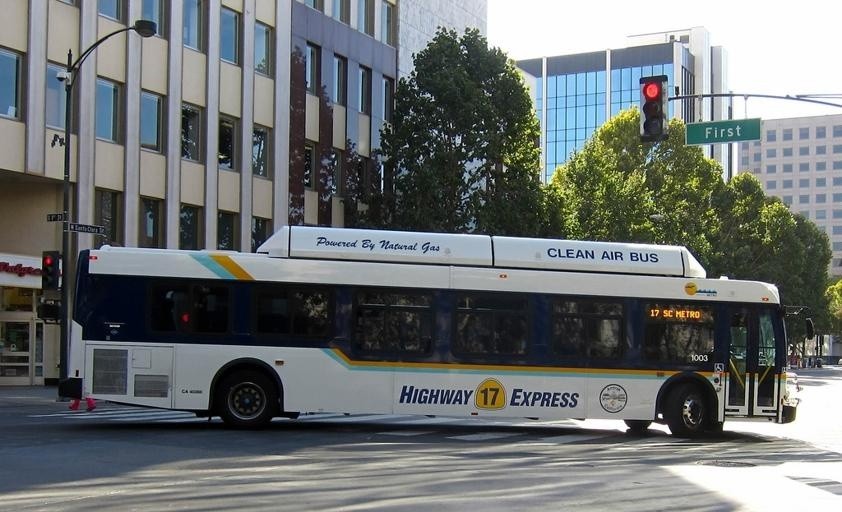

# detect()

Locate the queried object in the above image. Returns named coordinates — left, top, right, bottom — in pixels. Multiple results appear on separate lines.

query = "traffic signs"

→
left=69, top=223, right=104, bottom=235
left=684, top=118, right=762, bottom=147
left=46, top=214, right=64, bottom=222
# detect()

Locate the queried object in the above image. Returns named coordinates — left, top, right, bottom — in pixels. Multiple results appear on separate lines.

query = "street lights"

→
left=59, top=21, right=156, bottom=397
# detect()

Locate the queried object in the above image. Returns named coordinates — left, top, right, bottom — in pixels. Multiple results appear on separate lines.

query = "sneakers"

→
left=87, top=406, right=96, bottom=412
left=68, top=405, right=72, bottom=408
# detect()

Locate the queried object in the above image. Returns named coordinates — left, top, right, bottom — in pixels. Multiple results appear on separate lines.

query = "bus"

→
left=66, top=227, right=816, bottom=437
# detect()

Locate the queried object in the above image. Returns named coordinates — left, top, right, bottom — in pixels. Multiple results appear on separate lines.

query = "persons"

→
left=458, top=315, right=484, bottom=352
left=67, top=398, right=97, bottom=412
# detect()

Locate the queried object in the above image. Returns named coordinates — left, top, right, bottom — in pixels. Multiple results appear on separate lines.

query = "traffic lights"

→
left=41, top=250, right=58, bottom=291
left=637, top=75, right=671, bottom=143
